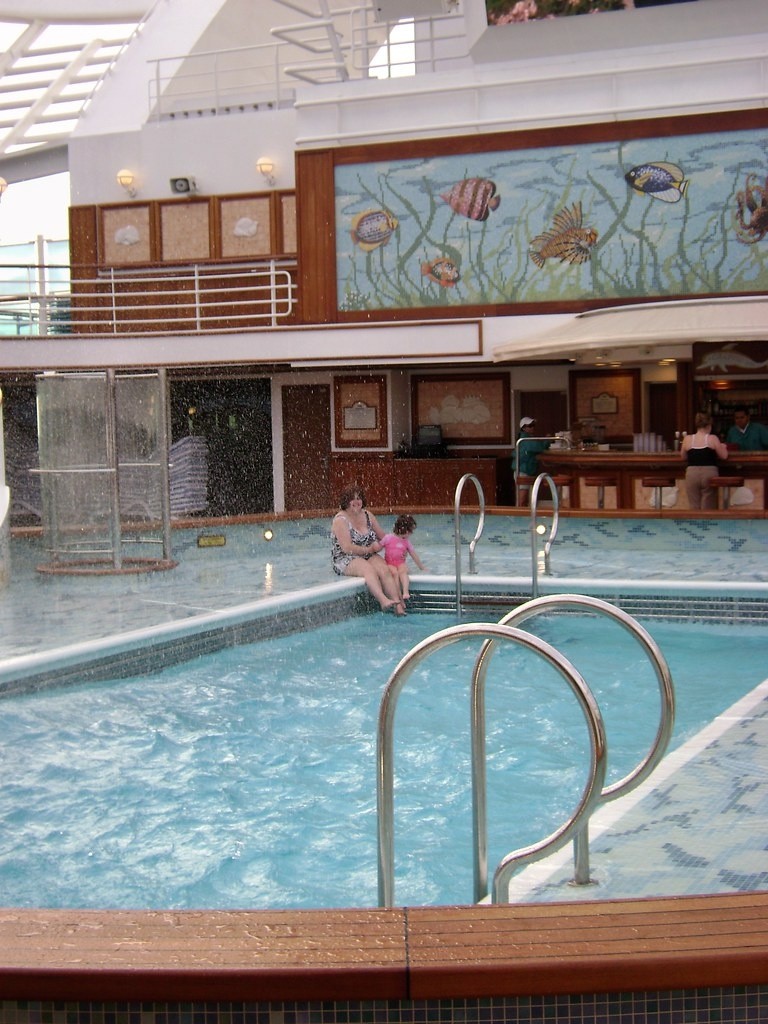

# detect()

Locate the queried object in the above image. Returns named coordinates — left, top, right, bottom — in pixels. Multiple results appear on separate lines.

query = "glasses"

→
left=526, top=425, right=535, bottom=428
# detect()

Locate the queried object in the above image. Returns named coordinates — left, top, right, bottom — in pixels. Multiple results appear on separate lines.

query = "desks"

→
left=537, top=449, right=768, bottom=509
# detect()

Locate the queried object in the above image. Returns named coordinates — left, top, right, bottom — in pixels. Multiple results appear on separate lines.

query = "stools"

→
left=706, top=477, right=745, bottom=510
left=585, top=478, right=618, bottom=509
left=517, top=474, right=575, bottom=508
left=642, top=477, right=675, bottom=510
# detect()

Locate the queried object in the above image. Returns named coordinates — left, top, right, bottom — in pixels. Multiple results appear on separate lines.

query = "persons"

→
left=681, top=412, right=729, bottom=510
left=372, top=514, right=428, bottom=610
left=725, top=405, right=768, bottom=451
left=330, top=490, right=409, bottom=616
left=510, top=416, right=554, bottom=507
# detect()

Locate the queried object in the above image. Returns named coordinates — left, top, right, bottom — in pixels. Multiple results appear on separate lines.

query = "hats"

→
left=520, top=417, right=536, bottom=429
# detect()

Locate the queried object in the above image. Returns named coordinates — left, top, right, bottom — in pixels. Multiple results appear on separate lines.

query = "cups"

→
left=586, top=443, right=599, bottom=450
left=633, top=433, right=666, bottom=453
